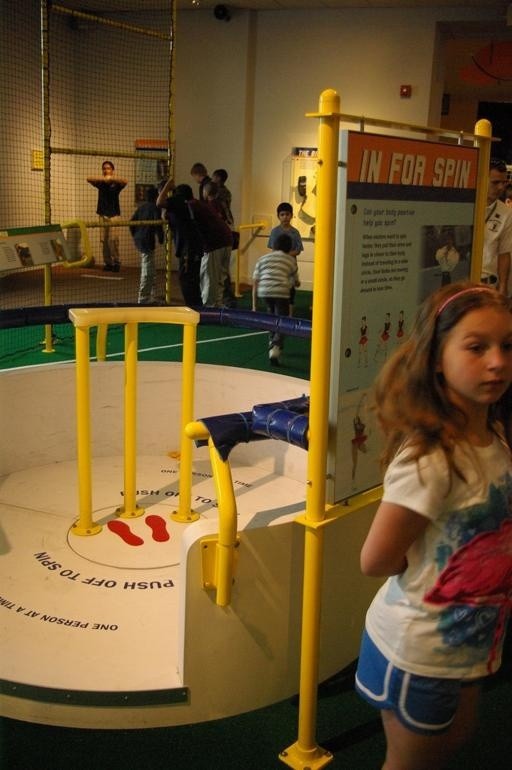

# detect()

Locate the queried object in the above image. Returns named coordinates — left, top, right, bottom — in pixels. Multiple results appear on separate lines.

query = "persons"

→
left=435, top=229, right=461, bottom=294
left=351, top=285, right=509, bottom=766
left=267, top=203, right=304, bottom=318
left=479, top=161, right=512, bottom=292
left=250, top=234, right=300, bottom=364
left=87, top=161, right=128, bottom=272
left=129, top=163, right=236, bottom=320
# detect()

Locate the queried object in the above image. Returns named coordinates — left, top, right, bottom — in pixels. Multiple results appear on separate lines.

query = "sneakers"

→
left=103, top=264, right=121, bottom=273
left=268, top=340, right=283, bottom=366
